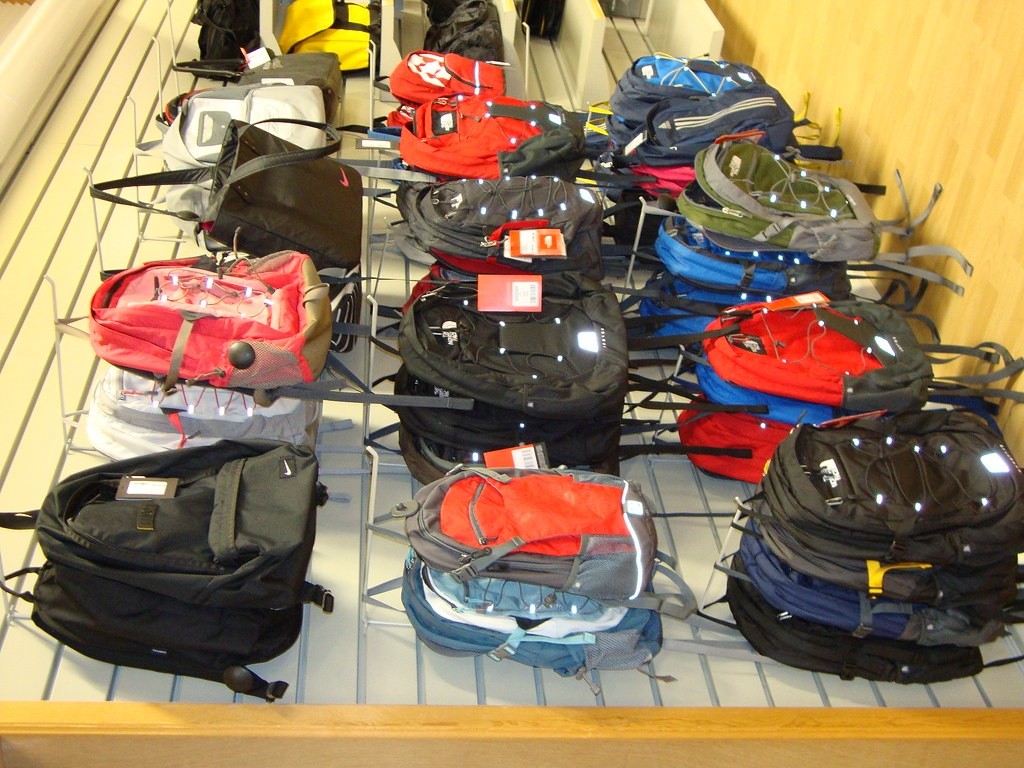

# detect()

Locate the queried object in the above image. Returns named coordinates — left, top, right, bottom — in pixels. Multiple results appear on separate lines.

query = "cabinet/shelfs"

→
left=256, top=0, right=728, bottom=107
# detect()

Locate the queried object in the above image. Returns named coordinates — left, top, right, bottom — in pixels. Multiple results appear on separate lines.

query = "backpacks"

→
left=402, top=547, right=664, bottom=678
left=406, top=464, right=656, bottom=603
left=583, top=53, right=1024, bottom=684
left=0, top=0, right=382, bottom=705
left=390, top=0, right=698, bottom=487
